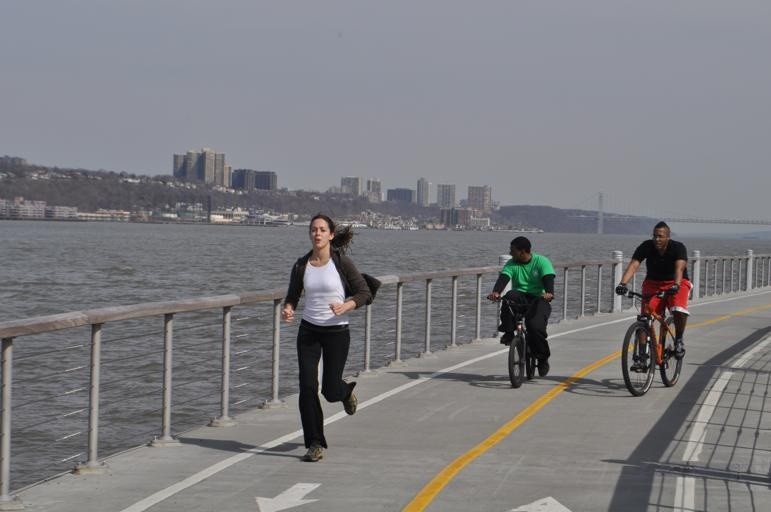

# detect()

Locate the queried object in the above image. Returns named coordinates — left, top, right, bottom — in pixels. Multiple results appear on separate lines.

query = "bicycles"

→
left=486, top=292, right=555, bottom=389
left=620, top=284, right=685, bottom=396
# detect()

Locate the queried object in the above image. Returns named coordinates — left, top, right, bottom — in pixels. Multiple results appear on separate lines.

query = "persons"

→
left=487, top=236, right=556, bottom=376
left=281, top=214, right=382, bottom=461
left=616, top=221, right=694, bottom=371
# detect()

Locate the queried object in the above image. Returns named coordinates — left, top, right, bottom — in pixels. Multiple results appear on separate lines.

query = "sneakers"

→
left=631, top=363, right=648, bottom=374
left=343, top=379, right=357, bottom=414
left=303, top=441, right=324, bottom=461
left=676, top=340, right=684, bottom=360
left=500, top=333, right=516, bottom=344
left=538, top=359, right=550, bottom=376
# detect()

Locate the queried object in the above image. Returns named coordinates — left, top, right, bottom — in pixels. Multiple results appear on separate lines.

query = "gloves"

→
left=667, top=284, right=679, bottom=295
left=615, top=282, right=628, bottom=295
